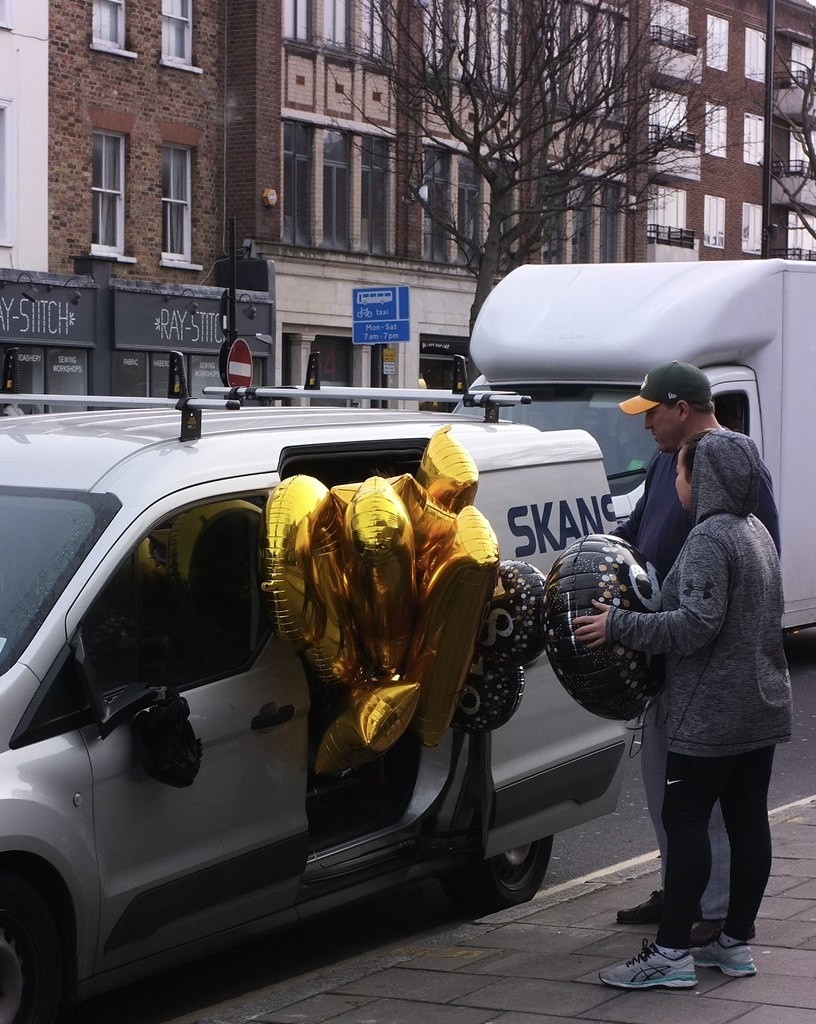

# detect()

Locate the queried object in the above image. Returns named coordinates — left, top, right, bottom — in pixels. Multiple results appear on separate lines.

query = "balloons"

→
left=111, top=501, right=266, bottom=659
left=451, top=556, right=555, bottom=732
left=260, top=418, right=502, bottom=788
left=540, top=532, right=669, bottom=719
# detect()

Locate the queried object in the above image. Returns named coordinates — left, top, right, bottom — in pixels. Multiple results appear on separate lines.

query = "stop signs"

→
left=225, top=337, right=254, bottom=388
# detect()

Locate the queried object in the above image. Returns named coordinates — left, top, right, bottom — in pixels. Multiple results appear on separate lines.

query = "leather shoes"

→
left=616, top=888, right=704, bottom=924
left=688, top=918, right=758, bottom=947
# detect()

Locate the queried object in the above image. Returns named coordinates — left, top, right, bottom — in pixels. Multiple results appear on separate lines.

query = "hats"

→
left=619, top=361, right=714, bottom=415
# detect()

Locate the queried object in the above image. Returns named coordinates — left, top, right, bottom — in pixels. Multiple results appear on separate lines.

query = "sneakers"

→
left=689, top=929, right=757, bottom=977
left=599, top=938, right=698, bottom=991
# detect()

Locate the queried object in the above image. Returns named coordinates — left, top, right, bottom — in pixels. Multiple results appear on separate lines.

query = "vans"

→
left=1, top=381, right=631, bottom=1024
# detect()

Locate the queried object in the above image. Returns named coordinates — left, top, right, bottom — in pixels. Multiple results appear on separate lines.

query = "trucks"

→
left=449, top=258, right=813, bottom=649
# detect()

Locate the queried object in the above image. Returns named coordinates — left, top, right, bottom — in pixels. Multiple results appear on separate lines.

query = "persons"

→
left=598, top=358, right=782, bottom=950
left=594, top=424, right=794, bottom=997
